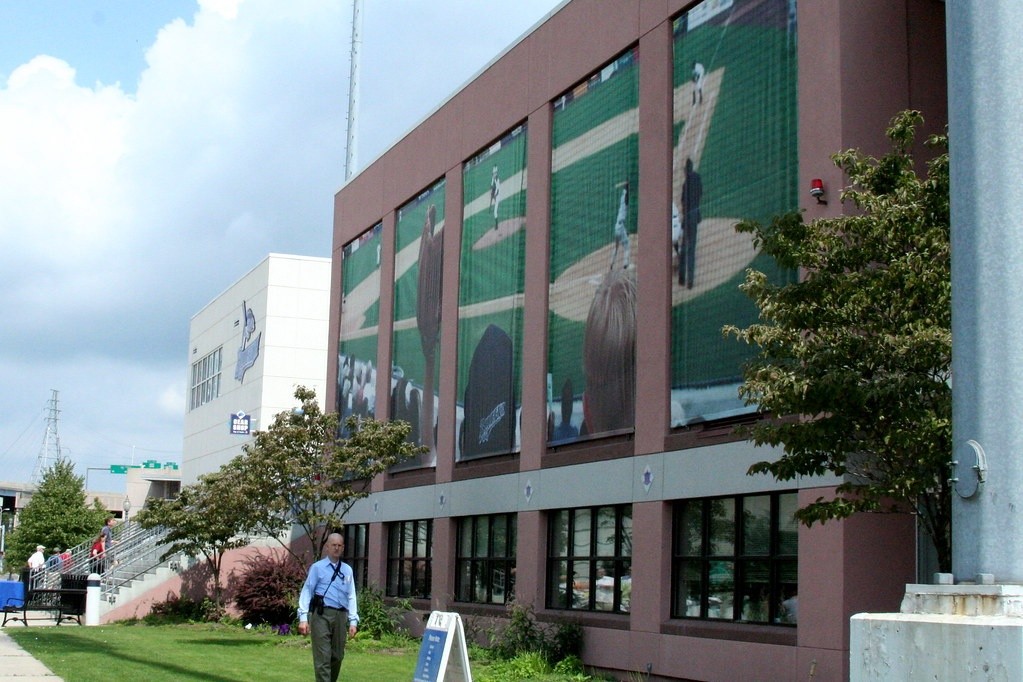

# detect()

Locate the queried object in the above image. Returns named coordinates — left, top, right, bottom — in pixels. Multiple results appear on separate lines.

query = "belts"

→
left=324, top=606, right=347, bottom=612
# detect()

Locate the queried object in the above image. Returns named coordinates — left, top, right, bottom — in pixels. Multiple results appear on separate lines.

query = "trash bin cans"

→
left=20, top=568, right=31, bottom=597
left=60, top=574, right=89, bottom=615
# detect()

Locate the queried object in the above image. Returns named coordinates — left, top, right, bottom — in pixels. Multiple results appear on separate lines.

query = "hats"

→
left=53, top=547, right=61, bottom=552
left=37, top=545, right=45, bottom=550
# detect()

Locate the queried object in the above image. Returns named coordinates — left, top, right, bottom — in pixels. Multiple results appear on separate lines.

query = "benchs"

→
left=2, top=587, right=88, bottom=627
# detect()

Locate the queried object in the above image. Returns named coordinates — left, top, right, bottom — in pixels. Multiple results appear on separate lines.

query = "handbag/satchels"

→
left=309, top=594, right=324, bottom=615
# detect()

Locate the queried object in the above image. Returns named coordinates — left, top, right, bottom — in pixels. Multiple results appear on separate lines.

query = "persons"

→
left=101, top=517, right=119, bottom=574
left=487, top=162, right=501, bottom=232
left=676, top=156, right=702, bottom=291
left=337, top=355, right=422, bottom=460
left=90, top=533, right=107, bottom=585
left=28, top=545, right=46, bottom=591
left=45, top=547, right=64, bottom=594
left=298, top=533, right=359, bottom=682
left=608, top=176, right=632, bottom=270
left=688, top=59, right=705, bottom=107
left=542, top=380, right=589, bottom=443
left=59, top=549, right=74, bottom=570
left=580, top=273, right=638, bottom=436
